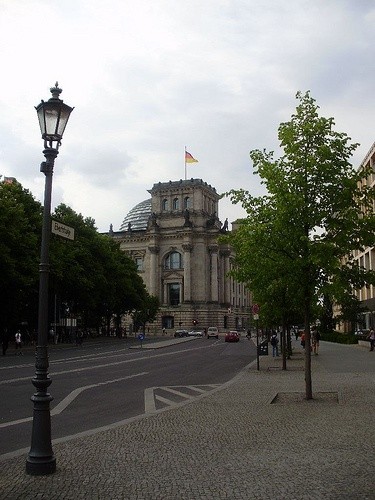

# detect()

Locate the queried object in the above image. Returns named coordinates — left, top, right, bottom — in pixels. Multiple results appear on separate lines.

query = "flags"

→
left=185, top=151, right=198, bottom=164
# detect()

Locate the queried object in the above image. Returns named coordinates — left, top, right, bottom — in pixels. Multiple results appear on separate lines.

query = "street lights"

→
left=24, top=81, right=76, bottom=477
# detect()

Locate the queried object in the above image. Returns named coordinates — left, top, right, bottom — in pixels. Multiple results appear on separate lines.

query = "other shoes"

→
left=276, top=355, right=279, bottom=356
left=370, top=350, right=373, bottom=351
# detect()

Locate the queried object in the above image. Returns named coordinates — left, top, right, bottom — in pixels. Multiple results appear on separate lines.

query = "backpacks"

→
left=271, top=336, right=277, bottom=346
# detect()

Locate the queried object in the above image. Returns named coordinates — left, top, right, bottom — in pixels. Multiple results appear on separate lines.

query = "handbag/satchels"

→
left=316, top=332, right=321, bottom=341
left=301, top=341, right=306, bottom=347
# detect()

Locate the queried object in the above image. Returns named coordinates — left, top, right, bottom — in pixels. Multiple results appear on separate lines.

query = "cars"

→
left=224, top=330, right=240, bottom=343
left=174, top=329, right=188, bottom=338
left=187, top=329, right=203, bottom=336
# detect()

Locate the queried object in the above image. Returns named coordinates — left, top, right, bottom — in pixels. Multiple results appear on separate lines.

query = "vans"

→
left=206, top=327, right=219, bottom=339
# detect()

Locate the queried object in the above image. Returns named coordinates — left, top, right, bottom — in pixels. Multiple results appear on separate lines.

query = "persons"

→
left=0, top=323, right=128, bottom=357
left=270, top=332, right=280, bottom=357
left=295, top=327, right=322, bottom=356
left=366, top=327, right=375, bottom=352
